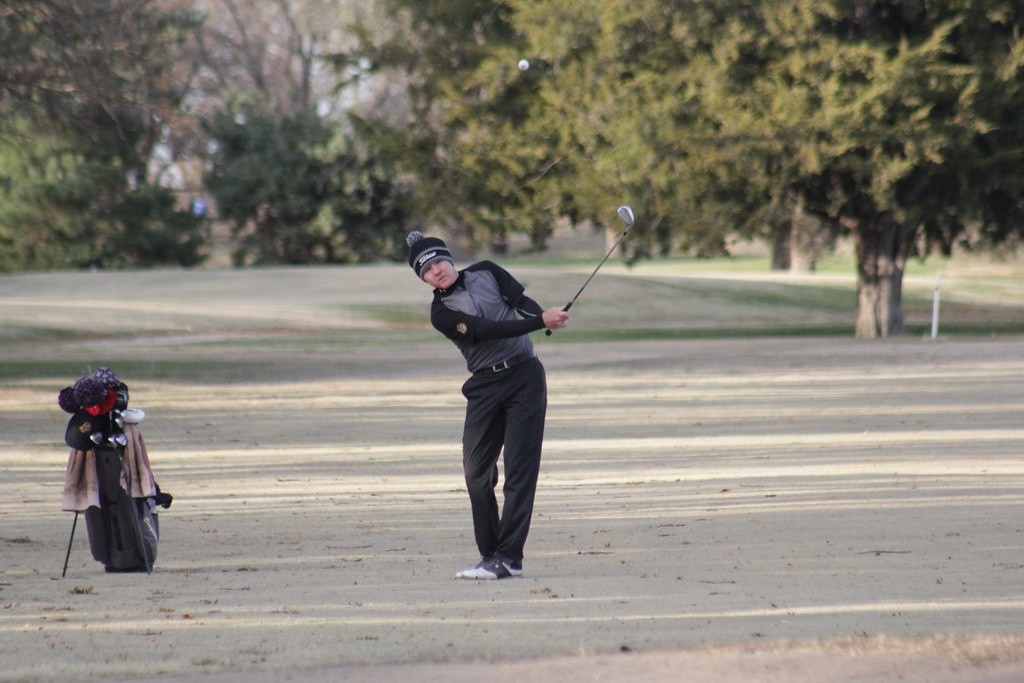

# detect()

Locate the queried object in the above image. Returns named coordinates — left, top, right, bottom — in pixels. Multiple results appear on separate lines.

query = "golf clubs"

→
left=85, top=391, right=127, bottom=456
left=545, top=205, right=635, bottom=336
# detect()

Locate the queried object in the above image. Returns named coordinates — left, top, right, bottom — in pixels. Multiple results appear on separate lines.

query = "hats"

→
left=407, top=230, right=454, bottom=283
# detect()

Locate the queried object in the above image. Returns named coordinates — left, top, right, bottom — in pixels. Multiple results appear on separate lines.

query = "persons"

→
left=400, top=229, right=570, bottom=580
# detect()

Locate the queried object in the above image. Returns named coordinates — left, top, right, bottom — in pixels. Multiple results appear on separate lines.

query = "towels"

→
left=61, top=416, right=157, bottom=514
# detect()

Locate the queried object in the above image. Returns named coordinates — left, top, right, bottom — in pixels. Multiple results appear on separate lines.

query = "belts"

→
left=475, top=350, right=536, bottom=375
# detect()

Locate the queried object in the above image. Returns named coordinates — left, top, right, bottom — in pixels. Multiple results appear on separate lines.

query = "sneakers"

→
left=455, top=558, right=522, bottom=581
